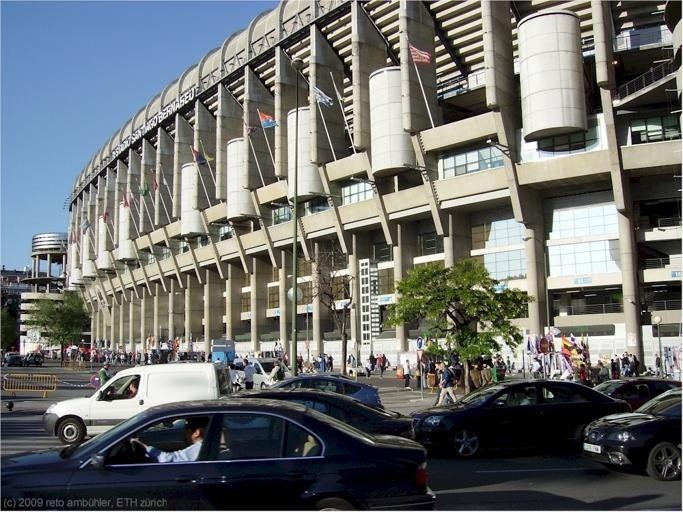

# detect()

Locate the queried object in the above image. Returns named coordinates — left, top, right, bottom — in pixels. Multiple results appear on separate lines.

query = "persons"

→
left=97, top=360, right=112, bottom=387
left=504, top=388, right=530, bottom=405
left=226, top=351, right=390, bottom=393
left=11, top=339, right=222, bottom=367
left=401, top=348, right=506, bottom=407
left=106, top=379, right=138, bottom=399
left=576, top=351, right=662, bottom=388
left=273, top=342, right=278, bottom=351
left=277, top=337, right=281, bottom=351
left=128, top=417, right=208, bottom=463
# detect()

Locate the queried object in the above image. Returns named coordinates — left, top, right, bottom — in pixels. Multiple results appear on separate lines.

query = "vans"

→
left=228, top=358, right=290, bottom=394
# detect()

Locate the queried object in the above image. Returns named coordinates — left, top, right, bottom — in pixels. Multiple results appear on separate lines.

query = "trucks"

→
left=42, top=361, right=217, bottom=451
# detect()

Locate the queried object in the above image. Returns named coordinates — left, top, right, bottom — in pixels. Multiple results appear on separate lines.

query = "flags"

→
left=64, top=164, right=169, bottom=247
left=311, top=84, right=334, bottom=110
left=190, top=147, right=207, bottom=166
left=199, top=140, right=214, bottom=162
left=559, top=333, right=591, bottom=369
left=331, top=77, right=345, bottom=104
left=408, top=43, right=432, bottom=64
left=257, top=111, right=278, bottom=130
left=243, top=121, right=261, bottom=138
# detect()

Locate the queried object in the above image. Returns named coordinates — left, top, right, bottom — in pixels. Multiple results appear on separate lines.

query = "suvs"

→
left=0, top=348, right=70, bottom=368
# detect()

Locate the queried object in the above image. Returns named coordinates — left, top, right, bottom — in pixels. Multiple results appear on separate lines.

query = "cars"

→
left=215, top=373, right=680, bottom=487
left=2, top=396, right=433, bottom=508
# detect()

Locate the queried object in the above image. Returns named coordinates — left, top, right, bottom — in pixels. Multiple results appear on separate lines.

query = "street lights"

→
left=518, top=233, right=555, bottom=376
left=284, top=273, right=311, bottom=373
left=652, top=316, right=663, bottom=377
